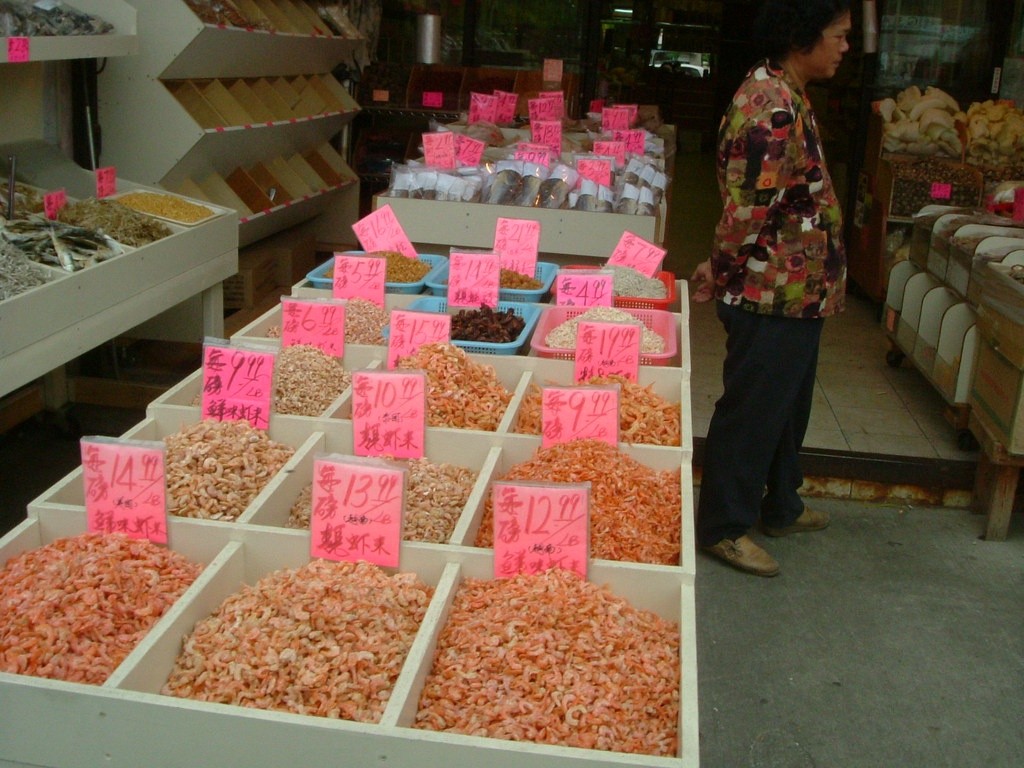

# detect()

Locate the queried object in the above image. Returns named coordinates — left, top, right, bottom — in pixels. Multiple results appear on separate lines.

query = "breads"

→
left=880, top=83, right=1024, bottom=167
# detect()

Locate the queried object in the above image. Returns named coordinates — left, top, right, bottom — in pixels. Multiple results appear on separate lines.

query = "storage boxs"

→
left=222, top=228, right=319, bottom=309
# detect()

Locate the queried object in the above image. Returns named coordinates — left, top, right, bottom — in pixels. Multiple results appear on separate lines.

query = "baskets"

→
left=529, top=304, right=677, bottom=366
left=382, top=296, right=542, bottom=356
left=306, top=249, right=447, bottom=295
left=551, top=265, right=675, bottom=310
left=424, top=256, right=560, bottom=301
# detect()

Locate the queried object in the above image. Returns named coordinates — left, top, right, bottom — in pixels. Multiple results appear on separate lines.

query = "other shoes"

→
left=758, top=506, right=832, bottom=538
left=697, top=534, right=781, bottom=578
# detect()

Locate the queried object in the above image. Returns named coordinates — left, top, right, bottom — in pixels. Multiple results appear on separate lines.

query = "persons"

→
left=935, top=61, right=978, bottom=113
left=689, top=0, right=852, bottom=575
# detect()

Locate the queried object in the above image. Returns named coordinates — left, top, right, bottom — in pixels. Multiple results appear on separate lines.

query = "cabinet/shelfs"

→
left=0, top=0, right=369, bottom=432
left=345, top=62, right=575, bottom=210
